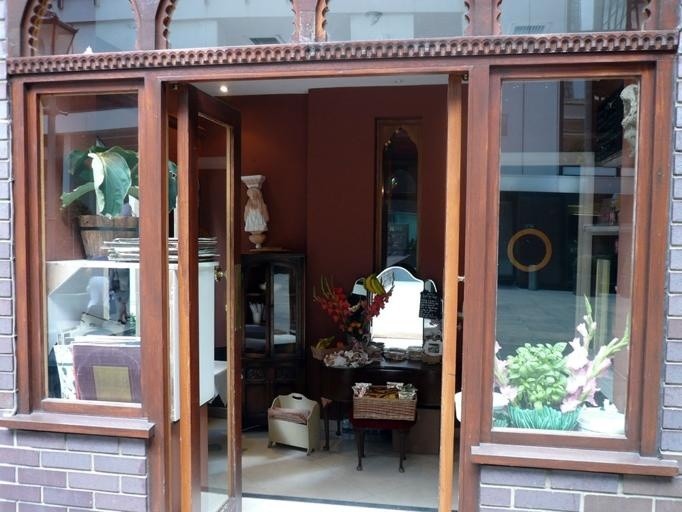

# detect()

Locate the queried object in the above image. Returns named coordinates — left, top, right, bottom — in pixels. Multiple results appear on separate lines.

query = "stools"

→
left=352, top=418, right=419, bottom=474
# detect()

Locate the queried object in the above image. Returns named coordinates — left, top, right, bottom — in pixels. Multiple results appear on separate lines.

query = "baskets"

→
left=353, top=394, right=417, bottom=421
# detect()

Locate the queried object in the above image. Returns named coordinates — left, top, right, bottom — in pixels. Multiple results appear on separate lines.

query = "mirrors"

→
left=366, top=266, right=424, bottom=349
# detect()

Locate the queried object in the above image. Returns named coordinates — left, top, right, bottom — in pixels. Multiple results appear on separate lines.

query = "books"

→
left=53, top=331, right=142, bottom=405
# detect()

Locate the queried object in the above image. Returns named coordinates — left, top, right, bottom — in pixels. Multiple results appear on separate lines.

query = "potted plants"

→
left=58, top=135, right=177, bottom=259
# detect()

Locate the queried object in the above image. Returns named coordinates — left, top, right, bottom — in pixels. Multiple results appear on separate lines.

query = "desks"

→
left=301, top=346, right=442, bottom=452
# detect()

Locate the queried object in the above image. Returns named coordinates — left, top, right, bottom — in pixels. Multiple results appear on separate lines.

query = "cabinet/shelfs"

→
left=237, top=248, right=308, bottom=429
left=45, top=258, right=219, bottom=422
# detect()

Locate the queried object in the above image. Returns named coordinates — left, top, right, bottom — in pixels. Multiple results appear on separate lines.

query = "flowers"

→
left=307, top=269, right=394, bottom=336
left=454, top=283, right=630, bottom=439
